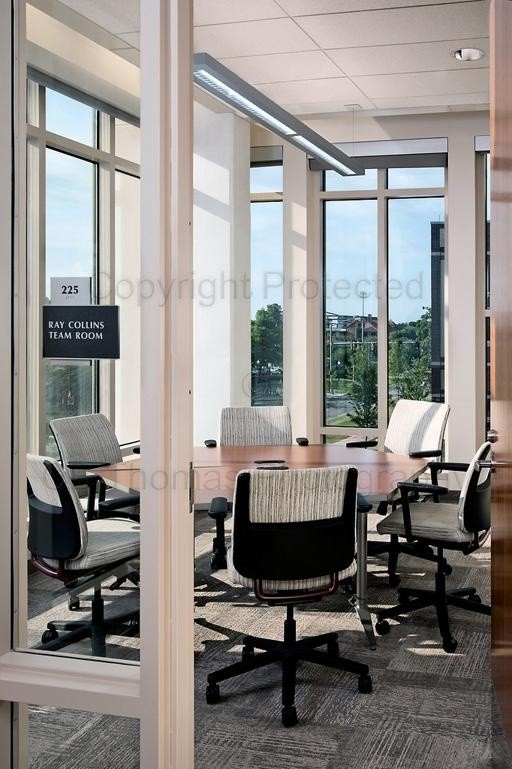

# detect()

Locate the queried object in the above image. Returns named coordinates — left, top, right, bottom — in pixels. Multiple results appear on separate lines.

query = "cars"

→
left=252, top=367, right=283, bottom=376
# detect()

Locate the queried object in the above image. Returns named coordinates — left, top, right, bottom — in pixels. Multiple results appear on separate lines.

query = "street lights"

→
left=359, top=290, right=369, bottom=315
left=327, top=322, right=334, bottom=394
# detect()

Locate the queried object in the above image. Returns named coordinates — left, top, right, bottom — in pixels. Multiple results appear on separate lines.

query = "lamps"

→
left=191, top=52, right=365, bottom=177
